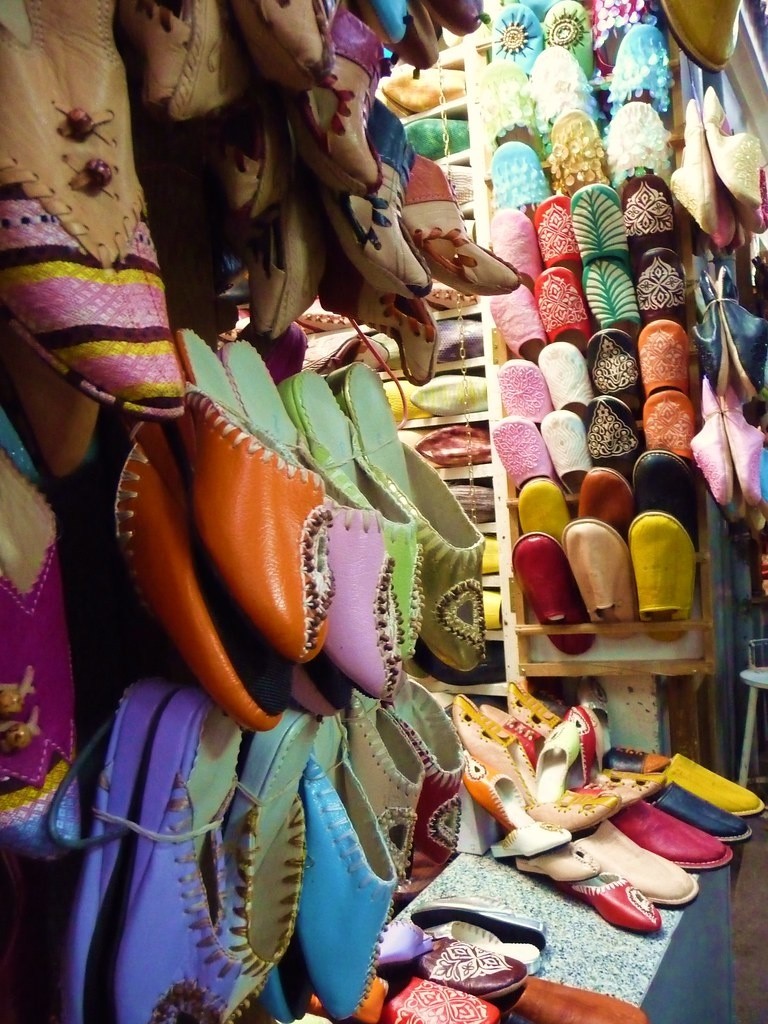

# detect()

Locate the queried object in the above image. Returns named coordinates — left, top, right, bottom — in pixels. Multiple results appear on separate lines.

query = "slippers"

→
left=0, top=0, right=768, bottom=1024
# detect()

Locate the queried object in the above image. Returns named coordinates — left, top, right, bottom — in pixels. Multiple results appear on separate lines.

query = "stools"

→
left=734, top=669, right=767, bottom=827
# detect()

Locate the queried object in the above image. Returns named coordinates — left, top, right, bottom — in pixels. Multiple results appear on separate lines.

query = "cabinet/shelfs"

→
left=307, top=2, right=768, bottom=779
